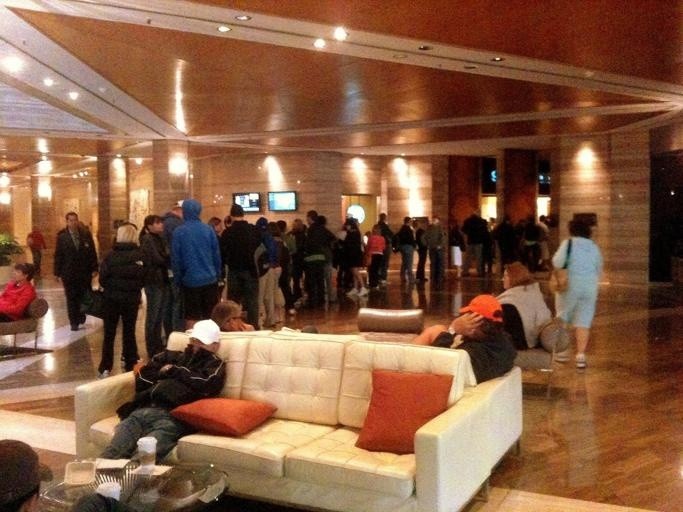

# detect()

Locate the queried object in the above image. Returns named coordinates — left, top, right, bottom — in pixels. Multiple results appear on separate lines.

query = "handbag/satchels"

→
left=80, top=282, right=105, bottom=319
left=548, top=238, right=572, bottom=292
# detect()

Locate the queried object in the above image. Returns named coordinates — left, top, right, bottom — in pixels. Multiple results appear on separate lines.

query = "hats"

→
left=457, top=293, right=505, bottom=325
left=189, top=319, right=222, bottom=346
left=231, top=204, right=244, bottom=217
left=210, top=300, right=243, bottom=327
left=1, top=440, right=54, bottom=504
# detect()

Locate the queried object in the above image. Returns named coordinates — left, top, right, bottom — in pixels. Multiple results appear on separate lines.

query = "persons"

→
left=54, top=212, right=99, bottom=330
left=417, top=220, right=428, bottom=283
left=396, top=216, right=416, bottom=283
left=336, top=218, right=369, bottom=297
left=139, top=215, right=171, bottom=356
left=431, top=216, right=446, bottom=279
left=208, top=217, right=226, bottom=290
left=375, top=213, right=395, bottom=285
left=463, top=207, right=489, bottom=277
left=451, top=221, right=466, bottom=280
left=0, top=439, right=121, bottom=512
left=26, top=227, right=46, bottom=273
left=171, top=198, right=222, bottom=321
left=255, top=208, right=337, bottom=329
left=1, top=262, right=35, bottom=323
left=366, top=225, right=385, bottom=287
left=495, top=262, right=552, bottom=350
left=488, top=213, right=552, bottom=277
left=551, top=220, right=604, bottom=369
left=410, top=294, right=515, bottom=383
left=221, top=205, right=261, bottom=330
left=99, top=320, right=227, bottom=465
left=99, top=224, right=144, bottom=375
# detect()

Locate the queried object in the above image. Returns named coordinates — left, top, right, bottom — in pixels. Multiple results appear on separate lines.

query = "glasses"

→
left=23, top=263, right=32, bottom=274
left=227, top=311, right=249, bottom=323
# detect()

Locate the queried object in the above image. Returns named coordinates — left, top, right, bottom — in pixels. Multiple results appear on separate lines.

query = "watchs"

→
left=448, top=327, right=456, bottom=337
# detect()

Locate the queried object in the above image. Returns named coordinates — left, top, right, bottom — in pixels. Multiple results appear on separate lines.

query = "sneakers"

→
left=98, top=369, right=110, bottom=380
left=289, top=308, right=296, bottom=314
left=345, top=288, right=359, bottom=296
left=416, top=277, right=419, bottom=279
left=555, top=349, right=569, bottom=363
left=356, top=286, right=368, bottom=296
left=575, top=354, right=587, bottom=369
left=424, top=278, right=428, bottom=281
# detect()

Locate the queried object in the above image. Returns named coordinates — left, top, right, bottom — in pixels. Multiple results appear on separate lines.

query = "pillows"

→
left=356, top=369, right=453, bottom=454
left=170, top=397, right=277, bottom=437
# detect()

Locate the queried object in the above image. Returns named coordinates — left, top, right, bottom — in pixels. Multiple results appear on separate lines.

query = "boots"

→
left=400, top=272, right=407, bottom=287
left=407, top=272, right=420, bottom=283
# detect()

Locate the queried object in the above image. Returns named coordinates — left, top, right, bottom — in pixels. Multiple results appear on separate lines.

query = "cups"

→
left=137, top=436, right=158, bottom=466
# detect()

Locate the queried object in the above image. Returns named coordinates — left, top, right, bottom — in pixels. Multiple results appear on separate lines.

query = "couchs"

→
left=75, top=332, right=520, bottom=511
left=515, top=323, right=570, bottom=403
left=358, top=309, right=427, bottom=343
left=187, top=329, right=365, bottom=342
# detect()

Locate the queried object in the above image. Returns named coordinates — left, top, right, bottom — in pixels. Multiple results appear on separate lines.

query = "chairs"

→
left=0, top=297, right=48, bottom=353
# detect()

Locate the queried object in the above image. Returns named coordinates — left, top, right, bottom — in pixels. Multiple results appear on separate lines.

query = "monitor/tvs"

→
left=267, top=190, right=297, bottom=212
left=232, top=191, right=262, bottom=214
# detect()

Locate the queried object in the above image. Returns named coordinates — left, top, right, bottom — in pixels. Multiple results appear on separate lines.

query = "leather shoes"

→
left=72, top=323, right=79, bottom=332
left=80, top=315, right=87, bottom=323
